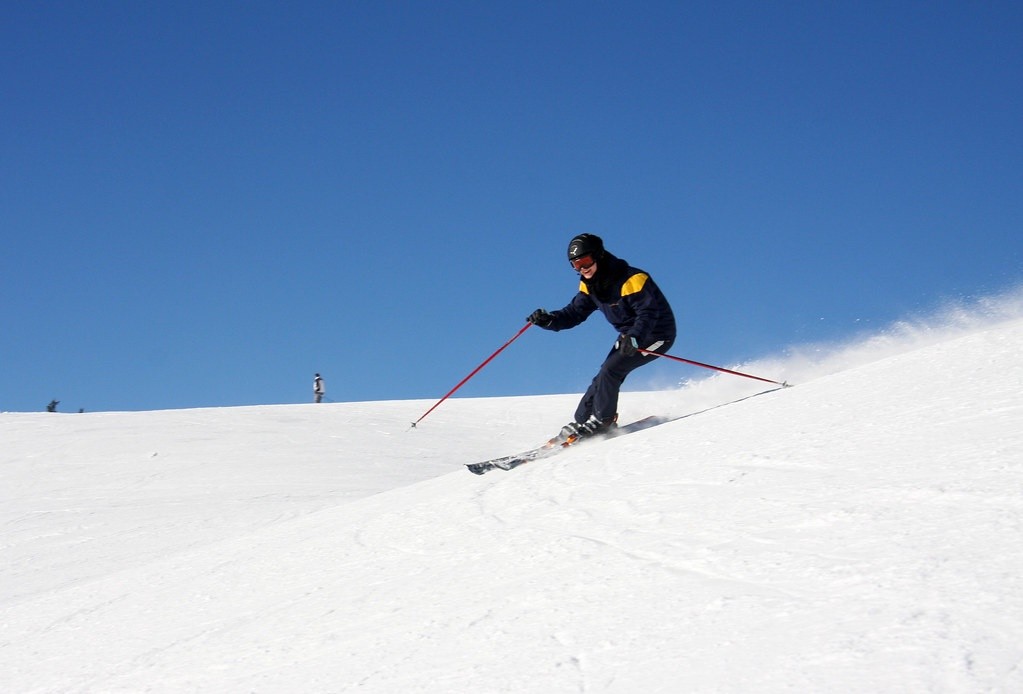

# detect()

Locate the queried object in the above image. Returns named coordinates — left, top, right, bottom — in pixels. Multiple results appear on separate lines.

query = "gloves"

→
left=526, top=308, right=555, bottom=328
left=615, top=336, right=638, bottom=357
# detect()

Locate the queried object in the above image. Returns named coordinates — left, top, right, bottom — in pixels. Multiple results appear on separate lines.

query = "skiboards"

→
left=462, top=416, right=670, bottom=475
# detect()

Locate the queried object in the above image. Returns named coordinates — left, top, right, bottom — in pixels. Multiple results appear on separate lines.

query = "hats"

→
left=567, top=233, right=604, bottom=261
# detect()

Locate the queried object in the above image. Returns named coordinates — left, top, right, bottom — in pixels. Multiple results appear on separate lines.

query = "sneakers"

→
left=578, top=418, right=608, bottom=436
left=561, top=421, right=579, bottom=435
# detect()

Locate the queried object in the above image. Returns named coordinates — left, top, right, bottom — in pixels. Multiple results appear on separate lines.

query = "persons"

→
left=47, top=399, right=61, bottom=412
left=526, top=233, right=677, bottom=437
left=313, top=373, right=325, bottom=403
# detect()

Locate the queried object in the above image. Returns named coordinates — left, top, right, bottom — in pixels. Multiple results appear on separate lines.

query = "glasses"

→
left=570, top=255, right=596, bottom=271
